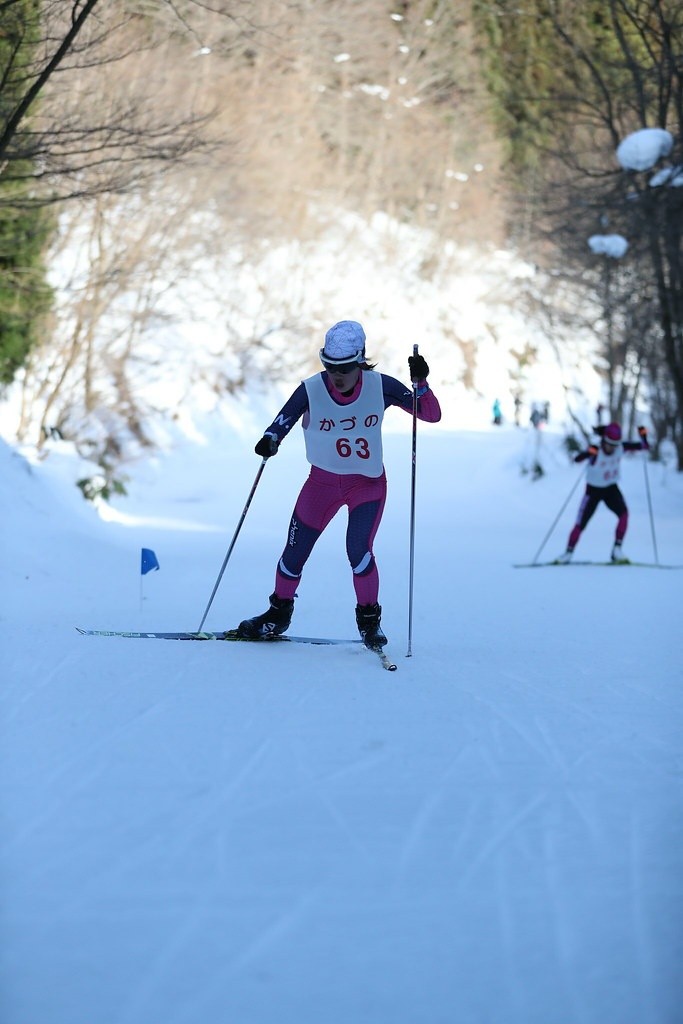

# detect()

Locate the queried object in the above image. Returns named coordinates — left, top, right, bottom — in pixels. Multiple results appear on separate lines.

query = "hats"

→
left=320, top=321, right=366, bottom=364
left=602, top=423, right=621, bottom=445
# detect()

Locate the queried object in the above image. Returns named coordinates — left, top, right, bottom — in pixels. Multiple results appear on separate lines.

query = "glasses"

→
left=323, top=361, right=359, bottom=374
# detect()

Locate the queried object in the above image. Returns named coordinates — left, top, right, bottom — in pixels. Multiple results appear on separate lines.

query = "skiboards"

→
left=72, top=624, right=400, bottom=672
left=511, top=560, right=683, bottom=570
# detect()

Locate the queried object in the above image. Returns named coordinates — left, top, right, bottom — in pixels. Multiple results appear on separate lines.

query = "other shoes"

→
left=554, top=550, right=572, bottom=566
left=611, top=544, right=629, bottom=563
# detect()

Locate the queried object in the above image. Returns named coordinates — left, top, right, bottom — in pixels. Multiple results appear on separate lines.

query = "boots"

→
left=237, top=593, right=294, bottom=638
left=355, top=603, right=387, bottom=647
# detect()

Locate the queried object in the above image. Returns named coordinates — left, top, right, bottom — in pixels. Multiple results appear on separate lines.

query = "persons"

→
left=491, top=396, right=550, bottom=429
left=555, top=422, right=650, bottom=565
left=240, top=320, right=441, bottom=648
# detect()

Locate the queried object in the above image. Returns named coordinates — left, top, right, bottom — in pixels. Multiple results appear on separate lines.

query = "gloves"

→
left=638, top=426, right=648, bottom=441
left=255, top=436, right=279, bottom=457
left=587, top=445, right=598, bottom=459
left=409, top=356, right=429, bottom=383
